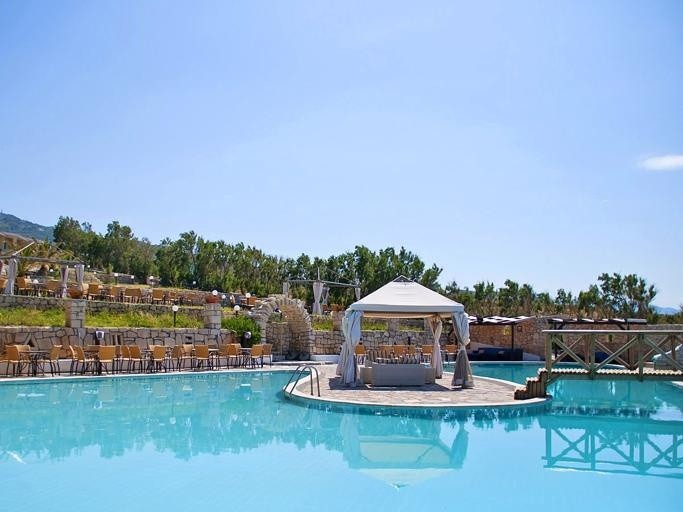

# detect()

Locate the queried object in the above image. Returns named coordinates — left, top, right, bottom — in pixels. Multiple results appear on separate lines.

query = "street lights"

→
left=171, top=305, right=178, bottom=327
left=233, top=305, right=240, bottom=317
left=210, top=289, right=251, bottom=307
left=73, top=263, right=153, bottom=287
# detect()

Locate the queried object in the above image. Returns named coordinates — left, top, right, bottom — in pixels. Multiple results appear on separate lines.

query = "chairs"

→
left=356, top=344, right=459, bottom=364
left=0, top=277, right=196, bottom=306
left=0, top=343, right=273, bottom=377
